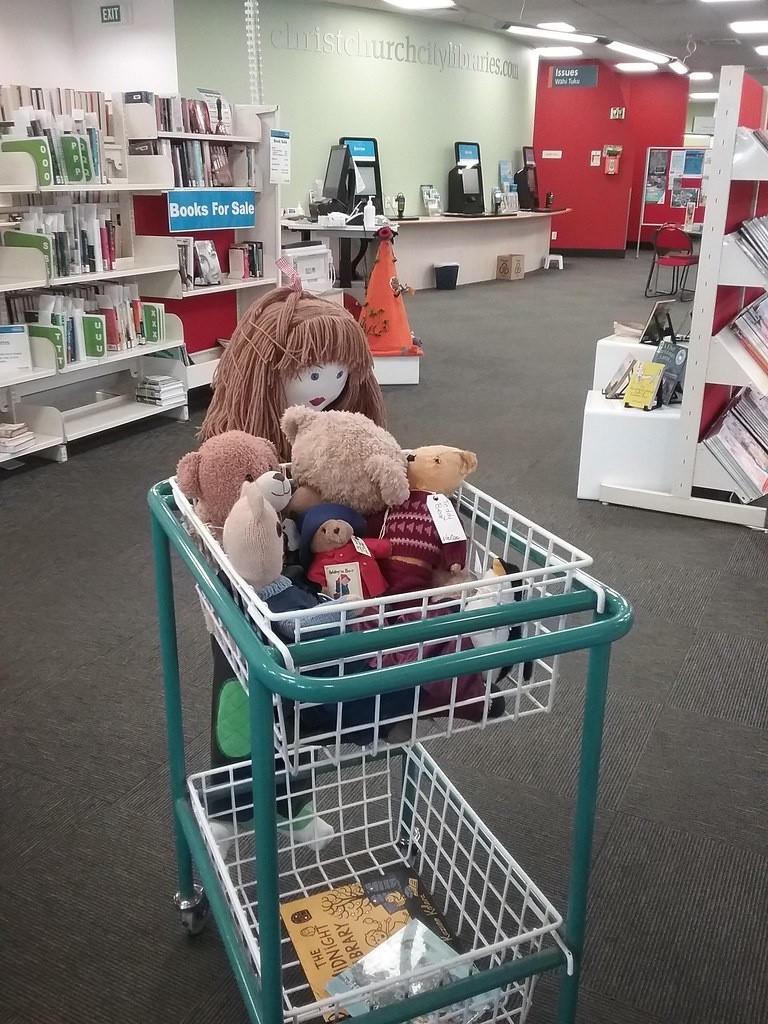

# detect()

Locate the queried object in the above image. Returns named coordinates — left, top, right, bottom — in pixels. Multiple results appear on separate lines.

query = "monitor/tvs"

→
left=323, top=145, right=357, bottom=217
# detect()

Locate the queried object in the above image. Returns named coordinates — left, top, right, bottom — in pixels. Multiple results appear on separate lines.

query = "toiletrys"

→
left=363, top=195, right=376, bottom=227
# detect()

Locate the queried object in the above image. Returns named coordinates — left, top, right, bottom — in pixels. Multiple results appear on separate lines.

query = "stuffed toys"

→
left=175, top=289, right=533, bottom=858
left=223, top=481, right=370, bottom=675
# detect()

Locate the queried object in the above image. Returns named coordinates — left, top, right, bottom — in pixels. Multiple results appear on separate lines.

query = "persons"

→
left=196, top=240, right=221, bottom=282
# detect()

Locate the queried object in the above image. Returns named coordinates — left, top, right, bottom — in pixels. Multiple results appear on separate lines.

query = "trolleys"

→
left=146, top=439, right=638, bottom=1024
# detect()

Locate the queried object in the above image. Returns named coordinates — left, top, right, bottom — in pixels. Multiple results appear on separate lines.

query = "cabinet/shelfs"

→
left=599, top=66, right=768, bottom=531
left=1, top=93, right=280, bottom=474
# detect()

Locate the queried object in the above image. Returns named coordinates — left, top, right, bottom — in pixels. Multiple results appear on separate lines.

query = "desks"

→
left=286, top=222, right=396, bottom=290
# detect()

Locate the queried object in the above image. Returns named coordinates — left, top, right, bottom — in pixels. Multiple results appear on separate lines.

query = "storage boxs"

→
left=496, top=254, right=525, bottom=279
left=281, top=247, right=333, bottom=286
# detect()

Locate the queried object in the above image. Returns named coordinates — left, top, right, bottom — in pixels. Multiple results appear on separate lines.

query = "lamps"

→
left=598, top=37, right=673, bottom=64
left=668, top=58, right=690, bottom=76
left=500, top=22, right=601, bottom=45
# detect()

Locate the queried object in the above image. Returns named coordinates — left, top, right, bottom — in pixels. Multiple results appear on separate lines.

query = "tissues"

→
left=318, top=211, right=351, bottom=226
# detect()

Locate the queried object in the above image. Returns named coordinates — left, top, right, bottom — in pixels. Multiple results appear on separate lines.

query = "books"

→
left=702, top=126, right=767, bottom=500
left=282, top=868, right=501, bottom=1024
left=1, top=84, right=186, bottom=451
left=171, top=97, right=262, bottom=290
left=605, top=300, right=694, bottom=406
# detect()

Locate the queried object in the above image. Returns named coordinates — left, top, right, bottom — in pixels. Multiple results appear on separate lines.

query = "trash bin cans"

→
left=434, top=262, right=459, bottom=290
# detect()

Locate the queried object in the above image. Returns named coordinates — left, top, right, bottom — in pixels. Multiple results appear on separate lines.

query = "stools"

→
left=544, top=254, right=564, bottom=269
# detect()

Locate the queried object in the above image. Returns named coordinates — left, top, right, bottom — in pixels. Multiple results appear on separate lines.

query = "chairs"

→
left=645, top=222, right=699, bottom=302
